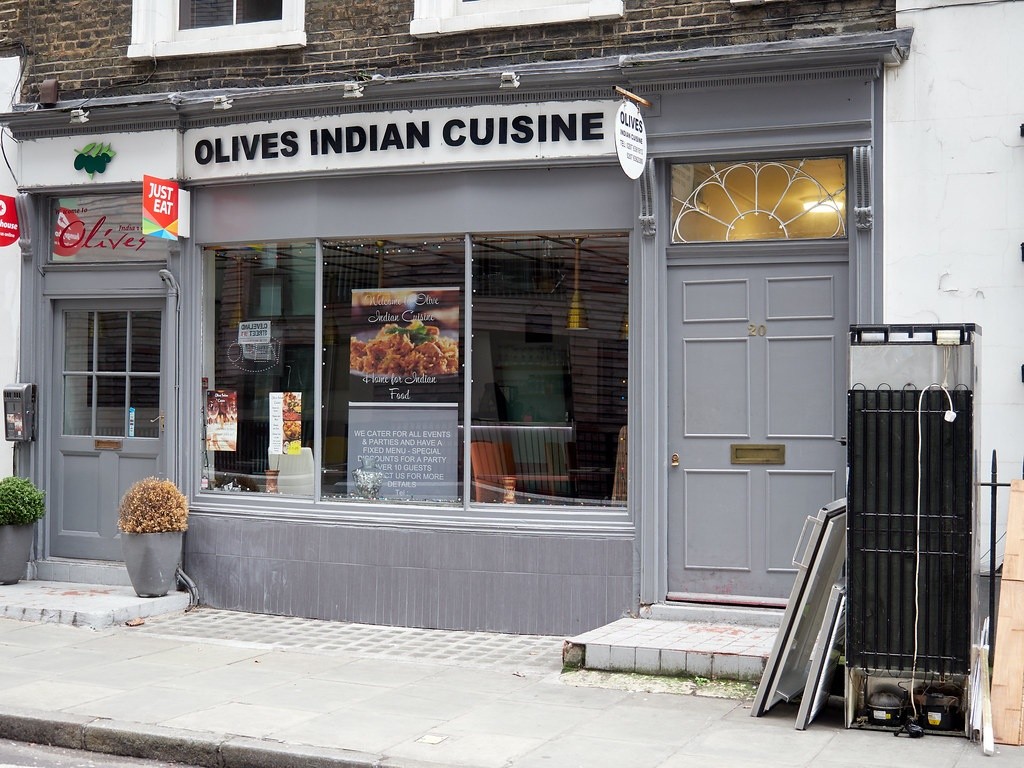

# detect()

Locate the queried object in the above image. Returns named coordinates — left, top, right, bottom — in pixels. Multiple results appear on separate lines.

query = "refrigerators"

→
left=840, top=320, right=985, bottom=740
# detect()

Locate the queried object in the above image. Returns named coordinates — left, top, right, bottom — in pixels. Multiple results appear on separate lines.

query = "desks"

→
left=473, top=474, right=616, bottom=480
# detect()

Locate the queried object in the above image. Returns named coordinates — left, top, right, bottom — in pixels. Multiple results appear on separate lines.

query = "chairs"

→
left=470, top=425, right=628, bottom=507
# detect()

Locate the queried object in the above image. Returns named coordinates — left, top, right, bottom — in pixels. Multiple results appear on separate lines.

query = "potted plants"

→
left=0, top=476, right=48, bottom=586
left=118, top=475, right=190, bottom=597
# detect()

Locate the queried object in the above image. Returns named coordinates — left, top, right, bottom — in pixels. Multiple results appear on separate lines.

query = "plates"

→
left=350, top=329, right=458, bottom=380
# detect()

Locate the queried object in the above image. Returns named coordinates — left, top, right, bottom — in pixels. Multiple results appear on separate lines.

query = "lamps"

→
left=566, top=238, right=588, bottom=331
left=619, top=313, right=628, bottom=339
left=799, top=195, right=845, bottom=213
left=213, top=94, right=234, bottom=111
left=343, top=84, right=364, bottom=99
left=498, top=72, right=521, bottom=89
left=70, top=110, right=91, bottom=124
